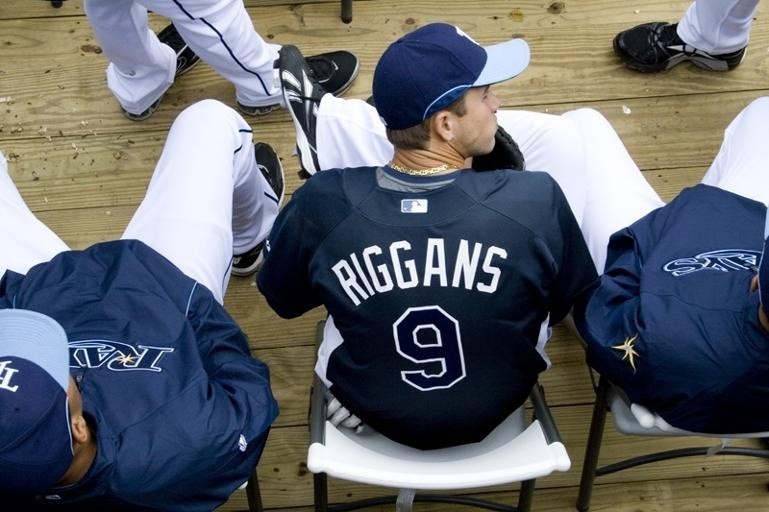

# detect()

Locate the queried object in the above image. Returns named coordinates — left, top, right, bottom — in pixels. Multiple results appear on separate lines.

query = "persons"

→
left=82, top=0, right=361, bottom=118
left=610, top=0, right=763, bottom=75
left=0, top=96, right=287, bottom=512
left=556, top=94, right=768, bottom=435
left=254, top=21, right=602, bottom=454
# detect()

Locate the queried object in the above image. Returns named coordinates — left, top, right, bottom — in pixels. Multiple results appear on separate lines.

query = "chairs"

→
left=576, top=347, right=766, bottom=512
left=308, top=321, right=570, bottom=511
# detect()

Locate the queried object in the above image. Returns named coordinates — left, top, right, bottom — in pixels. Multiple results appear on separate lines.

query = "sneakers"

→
left=614, top=18, right=746, bottom=72
left=230, top=141, right=286, bottom=277
left=278, top=44, right=327, bottom=176
left=118, top=22, right=201, bottom=120
left=235, top=49, right=357, bottom=115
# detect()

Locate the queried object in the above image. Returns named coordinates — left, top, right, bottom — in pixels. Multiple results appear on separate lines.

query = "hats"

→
left=1, top=307, right=74, bottom=488
left=373, top=23, right=531, bottom=129
